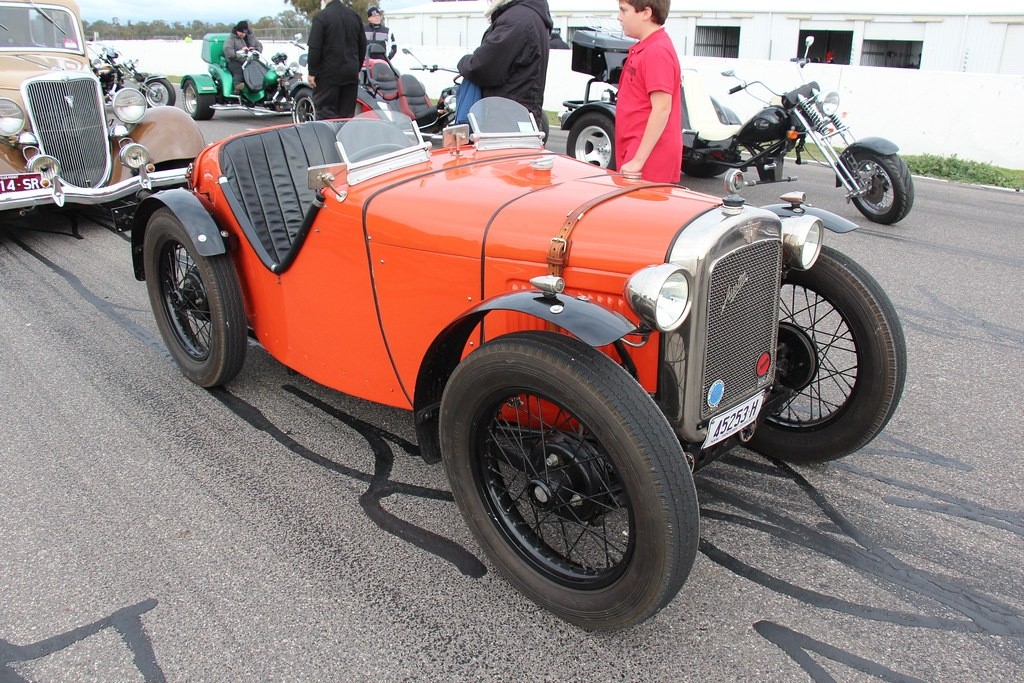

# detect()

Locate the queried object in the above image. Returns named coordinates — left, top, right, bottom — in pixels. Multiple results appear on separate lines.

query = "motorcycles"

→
left=130, top=96, right=910, bottom=637
left=179, top=32, right=303, bottom=121
left=289, top=44, right=463, bottom=135
left=87, top=45, right=176, bottom=108
left=557, top=28, right=915, bottom=226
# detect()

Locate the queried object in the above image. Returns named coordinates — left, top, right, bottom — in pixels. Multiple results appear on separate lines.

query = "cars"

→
left=0, top=0, right=206, bottom=218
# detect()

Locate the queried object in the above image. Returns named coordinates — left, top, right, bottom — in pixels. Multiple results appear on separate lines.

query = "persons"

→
left=363, top=6, right=397, bottom=60
left=614, top=0, right=682, bottom=184
left=224, top=21, right=263, bottom=94
left=457, top=0, right=553, bottom=132
left=307, top=0, right=367, bottom=121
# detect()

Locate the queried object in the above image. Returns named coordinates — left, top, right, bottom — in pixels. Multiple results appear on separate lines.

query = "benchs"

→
left=223, top=119, right=411, bottom=263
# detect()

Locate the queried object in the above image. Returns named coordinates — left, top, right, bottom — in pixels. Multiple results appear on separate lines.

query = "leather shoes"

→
left=233, top=82, right=245, bottom=95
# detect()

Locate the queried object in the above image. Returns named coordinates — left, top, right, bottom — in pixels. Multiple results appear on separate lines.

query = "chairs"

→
left=397, top=73, right=438, bottom=120
left=372, top=62, right=400, bottom=112
left=682, top=68, right=741, bottom=140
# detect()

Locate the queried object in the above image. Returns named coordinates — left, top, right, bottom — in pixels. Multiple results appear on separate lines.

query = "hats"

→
left=367, top=7, right=381, bottom=18
left=236, top=21, right=249, bottom=33
